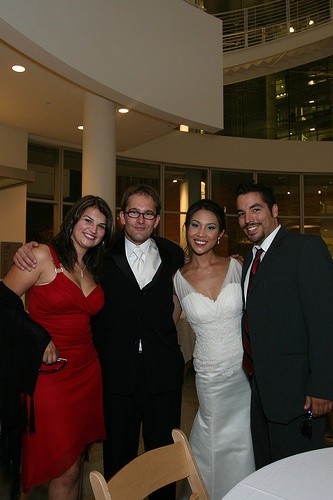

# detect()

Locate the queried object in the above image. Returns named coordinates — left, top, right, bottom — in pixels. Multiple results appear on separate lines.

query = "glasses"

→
left=124, top=210, right=157, bottom=220
left=29, top=357, right=67, bottom=375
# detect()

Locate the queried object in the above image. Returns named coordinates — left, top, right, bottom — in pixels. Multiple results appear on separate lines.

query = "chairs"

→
left=88, top=429, right=212, bottom=500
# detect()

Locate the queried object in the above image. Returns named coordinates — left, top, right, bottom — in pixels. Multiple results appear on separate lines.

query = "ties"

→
left=242, top=247, right=264, bottom=378
left=133, top=248, right=145, bottom=276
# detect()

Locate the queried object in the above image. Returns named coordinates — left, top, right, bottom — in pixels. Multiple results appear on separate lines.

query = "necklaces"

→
left=74, top=262, right=86, bottom=278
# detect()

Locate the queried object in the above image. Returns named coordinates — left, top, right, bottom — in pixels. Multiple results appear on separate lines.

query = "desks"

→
left=220, top=446, right=333, bottom=500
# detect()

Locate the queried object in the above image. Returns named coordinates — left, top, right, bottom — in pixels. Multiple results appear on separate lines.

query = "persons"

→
left=0, top=282, right=57, bottom=500
left=235, top=181, right=333, bottom=471
left=3, top=195, right=114, bottom=500
left=12, top=184, right=245, bottom=500
left=172, top=199, right=256, bottom=500
